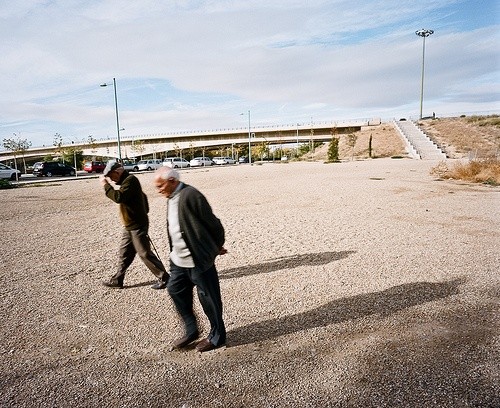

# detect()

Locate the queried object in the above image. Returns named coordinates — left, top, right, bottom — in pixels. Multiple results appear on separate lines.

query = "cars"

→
left=118, top=163, right=139, bottom=172
left=281, top=155, right=289, bottom=161
left=190, top=159, right=201, bottom=166
left=0, top=163, right=21, bottom=181
left=238, top=156, right=253, bottom=163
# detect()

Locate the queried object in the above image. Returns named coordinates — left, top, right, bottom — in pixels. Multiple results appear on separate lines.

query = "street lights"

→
left=240, top=110, right=251, bottom=164
left=100, top=78, right=122, bottom=162
left=415, top=28, right=434, bottom=119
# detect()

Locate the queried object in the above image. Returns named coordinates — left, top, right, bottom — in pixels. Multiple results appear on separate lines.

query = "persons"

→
left=154, top=167, right=227, bottom=353
left=99, top=162, right=170, bottom=290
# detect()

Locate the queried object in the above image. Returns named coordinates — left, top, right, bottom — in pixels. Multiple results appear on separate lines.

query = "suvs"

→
left=27, top=162, right=75, bottom=177
left=83, top=161, right=106, bottom=173
left=137, top=157, right=189, bottom=171
left=198, top=156, right=237, bottom=166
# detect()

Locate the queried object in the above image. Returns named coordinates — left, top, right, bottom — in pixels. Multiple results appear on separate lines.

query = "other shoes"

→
left=174, top=329, right=203, bottom=349
left=102, top=280, right=123, bottom=289
left=150, top=279, right=167, bottom=289
left=196, top=338, right=214, bottom=352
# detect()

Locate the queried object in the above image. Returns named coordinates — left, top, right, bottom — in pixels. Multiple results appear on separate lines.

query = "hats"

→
left=102, top=159, right=119, bottom=176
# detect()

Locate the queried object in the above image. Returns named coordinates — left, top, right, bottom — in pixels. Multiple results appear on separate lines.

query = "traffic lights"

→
left=251, top=133, right=255, bottom=138
